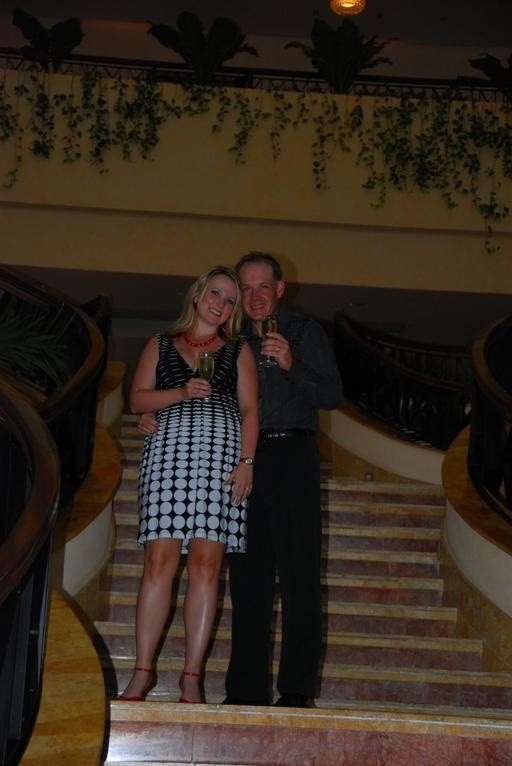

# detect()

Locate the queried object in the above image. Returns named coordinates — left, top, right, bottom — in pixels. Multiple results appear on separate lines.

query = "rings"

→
left=276, top=346, right=281, bottom=352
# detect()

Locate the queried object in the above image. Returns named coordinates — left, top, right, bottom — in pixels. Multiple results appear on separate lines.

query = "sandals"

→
left=177, top=671, right=202, bottom=703
left=115, top=667, right=157, bottom=700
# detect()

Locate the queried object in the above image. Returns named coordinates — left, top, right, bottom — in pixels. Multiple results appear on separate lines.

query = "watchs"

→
left=239, top=456, right=256, bottom=466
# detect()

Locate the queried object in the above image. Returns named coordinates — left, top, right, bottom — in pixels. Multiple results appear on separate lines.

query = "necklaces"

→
left=184, top=332, right=219, bottom=348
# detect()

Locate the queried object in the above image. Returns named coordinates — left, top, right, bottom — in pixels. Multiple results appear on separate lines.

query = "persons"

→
left=137, top=249, right=346, bottom=707
left=117, top=264, right=263, bottom=703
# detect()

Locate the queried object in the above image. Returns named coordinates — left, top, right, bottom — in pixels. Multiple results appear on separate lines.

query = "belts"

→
left=259, top=428, right=315, bottom=441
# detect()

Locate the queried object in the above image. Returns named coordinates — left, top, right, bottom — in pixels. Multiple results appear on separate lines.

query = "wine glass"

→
left=198, top=351, right=215, bottom=402
left=259, top=314, right=279, bottom=368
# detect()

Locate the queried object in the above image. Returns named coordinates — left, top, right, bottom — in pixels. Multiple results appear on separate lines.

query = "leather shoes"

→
left=271, top=696, right=317, bottom=708
left=218, top=695, right=274, bottom=707
